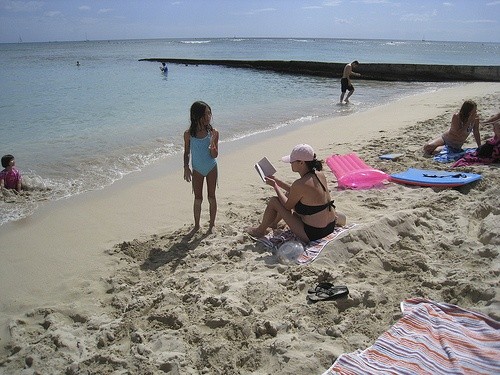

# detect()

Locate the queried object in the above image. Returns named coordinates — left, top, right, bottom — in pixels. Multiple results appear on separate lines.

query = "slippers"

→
left=308, top=282, right=349, bottom=302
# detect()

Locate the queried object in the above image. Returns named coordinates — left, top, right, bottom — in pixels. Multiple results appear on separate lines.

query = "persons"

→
left=0, top=154, right=22, bottom=193
left=339, top=59, right=362, bottom=104
left=423, top=100, right=482, bottom=155
left=183, top=101, right=220, bottom=234
left=246, top=144, right=337, bottom=244
left=160, top=61, right=168, bottom=75
left=486, top=122, right=500, bottom=159
left=76, top=60, right=80, bottom=67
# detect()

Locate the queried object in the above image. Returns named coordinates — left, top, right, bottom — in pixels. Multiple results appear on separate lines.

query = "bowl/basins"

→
left=276, top=241, right=305, bottom=265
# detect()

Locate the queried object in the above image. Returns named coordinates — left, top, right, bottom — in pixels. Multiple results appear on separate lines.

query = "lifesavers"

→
left=390, top=168, right=482, bottom=188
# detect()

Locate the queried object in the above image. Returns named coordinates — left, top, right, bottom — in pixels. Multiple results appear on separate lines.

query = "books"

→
left=254, top=156, right=278, bottom=181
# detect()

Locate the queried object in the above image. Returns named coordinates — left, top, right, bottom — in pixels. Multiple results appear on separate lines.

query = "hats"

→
left=281, top=143, right=316, bottom=163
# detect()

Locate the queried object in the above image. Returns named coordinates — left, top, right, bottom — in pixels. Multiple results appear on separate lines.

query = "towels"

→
left=450, top=138, right=500, bottom=170
left=243, top=220, right=357, bottom=264
left=431, top=144, right=477, bottom=163
left=319, top=298, right=500, bottom=375
